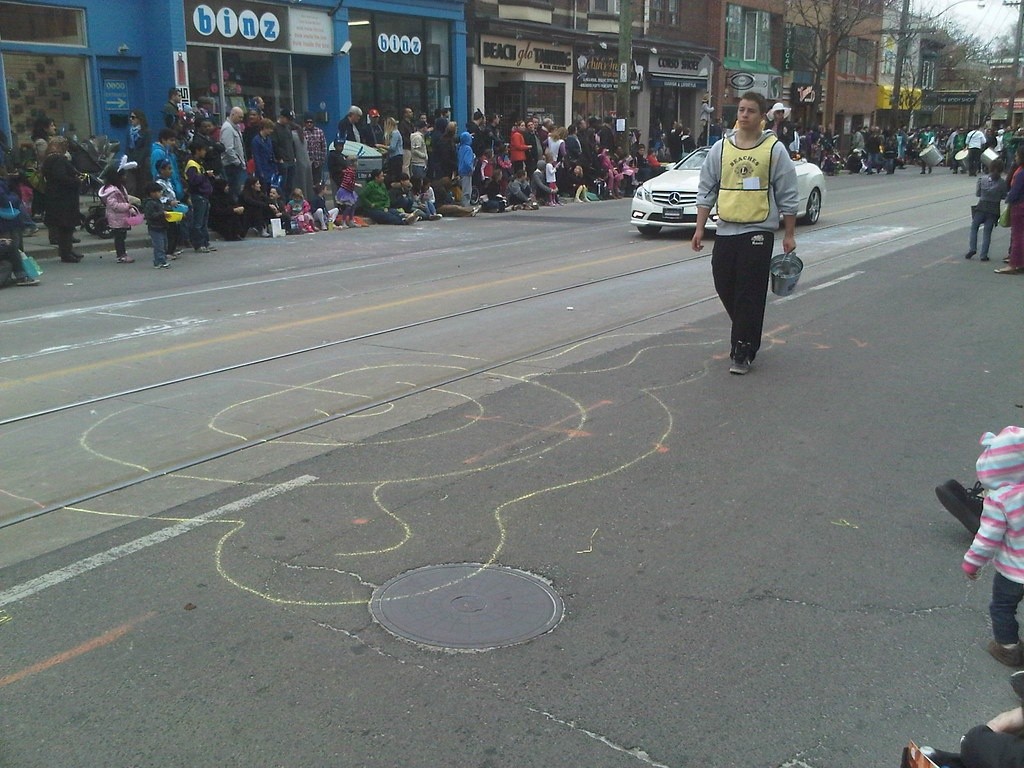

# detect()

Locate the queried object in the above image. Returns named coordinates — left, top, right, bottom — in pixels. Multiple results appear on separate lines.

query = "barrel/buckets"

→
left=955, top=149, right=971, bottom=170
left=918, top=144, right=944, bottom=167
left=979, top=147, right=1002, bottom=170
left=768, top=251, right=804, bottom=295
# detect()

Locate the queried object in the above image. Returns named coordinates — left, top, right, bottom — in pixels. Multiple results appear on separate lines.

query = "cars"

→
left=630, top=146, right=826, bottom=235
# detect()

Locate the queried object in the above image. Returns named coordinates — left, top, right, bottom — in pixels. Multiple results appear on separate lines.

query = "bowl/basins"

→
left=166, top=212, right=183, bottom=222
left=0, top=207, right=21, bottom=221
left=171, top=203, right=189, bottom=214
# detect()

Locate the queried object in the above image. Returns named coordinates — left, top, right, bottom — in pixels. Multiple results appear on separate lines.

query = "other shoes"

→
left=827, top=159, right=994, bottom=182
left=980, top=257, right=989, bottom=260
left=965, top=251, right=977, bottom=260
left=117, top=255, right=135, bottom=264
left=984, top=638, right=1022, bottom=666
left=71, top=236, right=81, bottom=243
left=15, top=276, right=41, bottom=286
left=993, top=254, right=1024, bottom=274
left=729, top=357, right=752, bottom=375
left=48, top=239, right=59, bottom=245
left=146, top=182, right=643, bottom=281
left=935, top=479, right=985, bottom=536
left=59, top=253, right=85, bottom=263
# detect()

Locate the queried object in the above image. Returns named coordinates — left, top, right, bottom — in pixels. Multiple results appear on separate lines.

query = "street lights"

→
left=887, top=0, right=988, bottom=137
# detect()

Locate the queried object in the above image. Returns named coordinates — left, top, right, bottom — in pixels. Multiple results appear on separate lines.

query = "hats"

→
left=334, top=137, right=345, bottom=147
left=702, top=94, right=713, bottom=102
left=281, top=108, right=294, bottom=120
left=766, top=102, right=792, bottom=122
left=368, top=109, right=380, bottom=118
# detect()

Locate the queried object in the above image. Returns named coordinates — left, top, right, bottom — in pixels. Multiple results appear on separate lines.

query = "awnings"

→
left=878, top=85, right=922, bottom=110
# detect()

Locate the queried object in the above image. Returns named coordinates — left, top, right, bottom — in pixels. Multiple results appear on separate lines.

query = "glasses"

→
left=130, top=115, right=137, bottom=119
left=306, top=121, right=313, bottom=123
left=776, top=110, right=785, bottom=114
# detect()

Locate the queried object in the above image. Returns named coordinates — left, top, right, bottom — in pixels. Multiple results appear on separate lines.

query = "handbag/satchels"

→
left=19, top=249, right=44, bottom=279
left=999, top=203, right=1011, bottom=228
left=971, top=205, right=984, bottom=224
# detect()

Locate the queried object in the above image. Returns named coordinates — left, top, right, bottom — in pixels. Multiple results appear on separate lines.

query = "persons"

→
left=700, top=94, right=714, bottom=129
left=966, top=160, right=1008, bottom=261
left=0, top=88, right=697, bottom=286
left=691, top=91, right=799, bottom=373
left=994, top=144, right=1024, bottom=274
left=702, top=114, right=737, bottom=146
left=903, top=423, right=1024, bottom=768
left=848, top=123, right=1024, bottom=176
left=761, top=102, right=836, bottom=177
